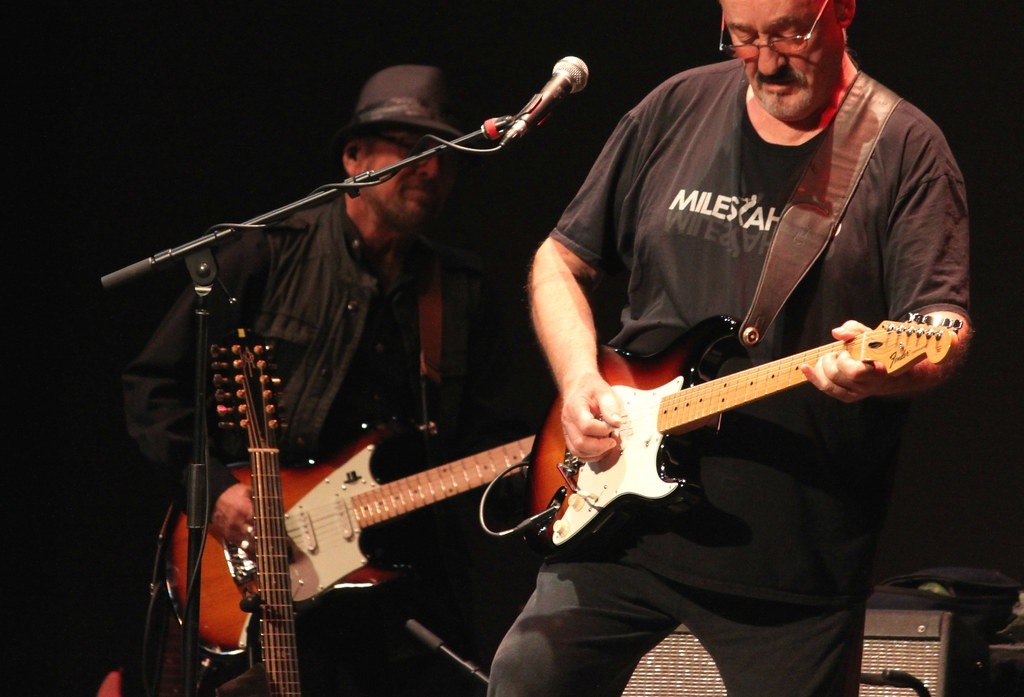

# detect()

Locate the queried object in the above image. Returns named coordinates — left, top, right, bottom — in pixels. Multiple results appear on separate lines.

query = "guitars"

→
left=519, top=312, right=965, bottom=553
left=164, top=428, right=535, bottom=657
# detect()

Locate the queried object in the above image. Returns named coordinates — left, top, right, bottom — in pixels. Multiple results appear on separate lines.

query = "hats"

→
left=329, top=66, right=469, bottom=138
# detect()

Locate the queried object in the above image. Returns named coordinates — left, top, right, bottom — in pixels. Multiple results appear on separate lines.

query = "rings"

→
left=247, top=526, right=253, bottom=535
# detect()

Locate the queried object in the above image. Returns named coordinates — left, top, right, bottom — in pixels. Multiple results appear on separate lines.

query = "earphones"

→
left=348, top=147, right=358, bottom=159
left=836, top=3, right=848, bottom=20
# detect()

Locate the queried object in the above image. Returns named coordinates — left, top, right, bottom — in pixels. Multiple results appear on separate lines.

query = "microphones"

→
left=500, top=56, right=588, bottom=147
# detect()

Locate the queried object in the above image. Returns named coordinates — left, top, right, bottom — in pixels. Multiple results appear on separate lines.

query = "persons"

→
left=487, top=0, right=971, bottom=697
left=119, top=67, right=490, bottom=679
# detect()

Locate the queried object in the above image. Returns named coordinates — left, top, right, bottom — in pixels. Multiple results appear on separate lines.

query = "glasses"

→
left=719, top=0, right=828, bottom=56
left=379, top=132, right=456, bottom=166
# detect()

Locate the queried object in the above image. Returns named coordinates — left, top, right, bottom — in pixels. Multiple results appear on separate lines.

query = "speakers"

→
left=621, top=608, right=991, bottom=697
